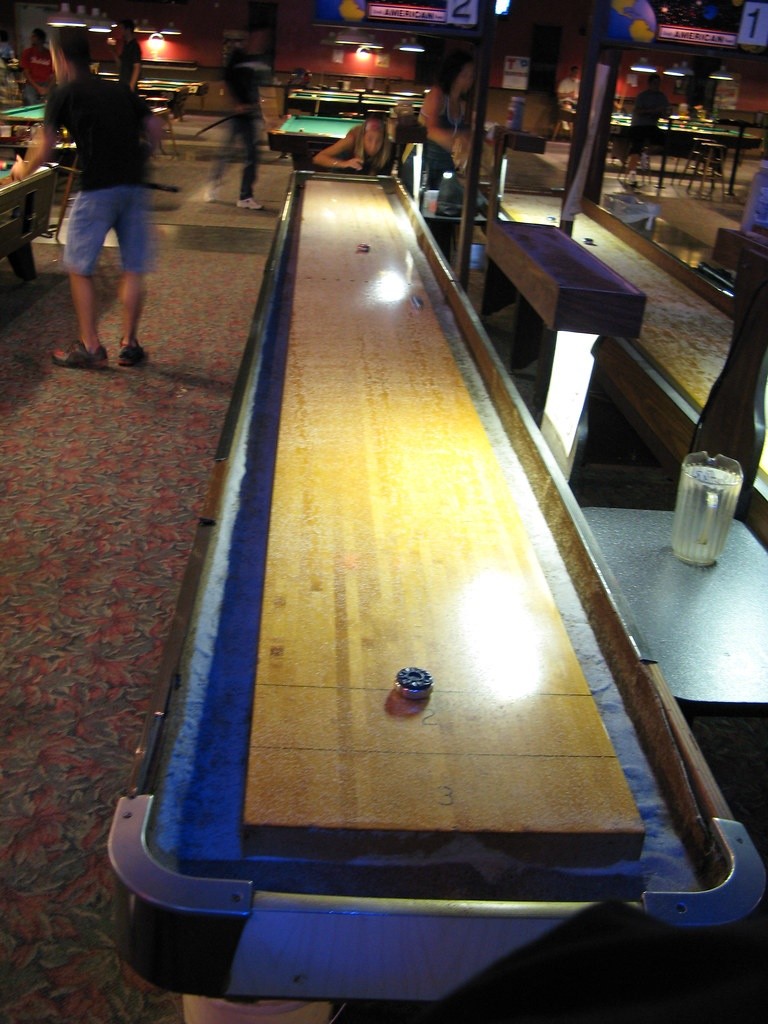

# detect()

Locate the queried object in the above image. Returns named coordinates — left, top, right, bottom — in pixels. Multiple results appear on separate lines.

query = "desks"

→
left=557, top=109, right=764, bottom=165
left=0, top=164, right=58, bottom=282
left=271, top=114, right=365, bottom=168
left=290, top=90, right=424, bottom=119
left=0, top=100, right=171, bottom=165
left=107, top=78, right=208, bottom=120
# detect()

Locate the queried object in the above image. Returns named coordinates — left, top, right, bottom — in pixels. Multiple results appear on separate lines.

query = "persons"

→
left=18, top=29, right=56, bottom=106
left=626, top=75, right=672, bottom=196
left=313, top=118, right=396, bottom=175
left=398, top=46, right=493, bottom=175
left=10, top=27, right=164, bottom=369
left=203, top=42, right=268, bottom=209
left=557, top=65, right=580, bottom=131
left=119, top=19, right=142, bottom=97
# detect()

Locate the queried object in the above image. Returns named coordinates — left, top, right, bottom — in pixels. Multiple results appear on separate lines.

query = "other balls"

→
left=393, top=666, right=434, bottom=700
left=357, top=243, right=370, bottom=252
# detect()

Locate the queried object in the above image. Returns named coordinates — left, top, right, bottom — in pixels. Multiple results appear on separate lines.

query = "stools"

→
left=678, top=136, right=726, bottom=197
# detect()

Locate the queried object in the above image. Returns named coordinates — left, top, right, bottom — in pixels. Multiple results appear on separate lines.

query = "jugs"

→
left=672, top=451, right=744, bottom=568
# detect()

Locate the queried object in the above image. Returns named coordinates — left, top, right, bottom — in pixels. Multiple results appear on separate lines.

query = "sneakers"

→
left=236, top=197, right=263, bottom=210
left=204, top=187, right=217, bottom=203
left=52, top=340, right=109, bottom=368
left=118, top=337, right=145, bottom=364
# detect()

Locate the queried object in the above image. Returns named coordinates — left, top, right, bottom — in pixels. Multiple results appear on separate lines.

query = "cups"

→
left=423, top=190, right=439, bottom=216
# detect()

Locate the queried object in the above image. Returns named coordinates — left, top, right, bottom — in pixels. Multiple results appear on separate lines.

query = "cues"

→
left=42, top=159, right=185, bottom=196
left=314, top=56, right=328, bottom=116
left=617, top=71, right=632, bottom=113
left=193, top=113, right=241, bottom=138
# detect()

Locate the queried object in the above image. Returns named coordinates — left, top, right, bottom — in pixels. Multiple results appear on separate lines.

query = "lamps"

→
left=134, top=19, right=158, bottom=34
left=630, top=57, right=657, bottom=73
left=393, top=38, right=424, bottom=52
left=680, top=62, right=693, bottom=77
left=335, top=29, right=371, bottom=45
left=160, top=1, right=181, bottom=34
left=46, top=3, right=118, bottom=33
left=708, top=67, right=734, bottom=81
left=362, top=37, right=384, bottom=49
left=663, top=63, right=685, bottom=77
